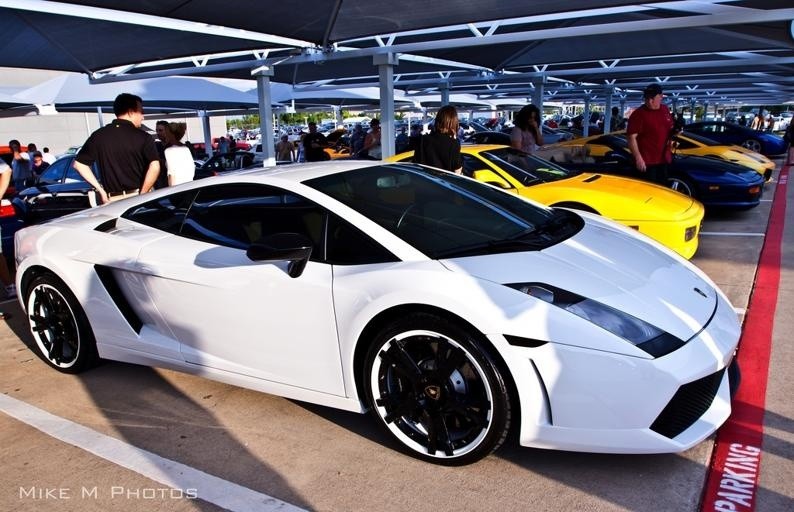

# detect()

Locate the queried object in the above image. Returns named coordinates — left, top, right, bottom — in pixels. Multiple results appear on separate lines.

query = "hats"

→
left=167, top=122, right=187, bottom=141
left=642, top=84, right=662, bottom=99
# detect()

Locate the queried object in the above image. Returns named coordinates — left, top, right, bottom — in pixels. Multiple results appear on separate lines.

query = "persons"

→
left=0, top=158, right=20, bottom=320
left=72, top=92, right=163, bottom=206
left=739, top=111, right=793, bottom=166
left=625, top=85, right=676, bottom=188
left=0, top=105, right=686, bottom=207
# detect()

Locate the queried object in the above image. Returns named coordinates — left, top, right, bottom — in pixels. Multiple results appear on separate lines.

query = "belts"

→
left=106, top=187, right=139, bottom=197
